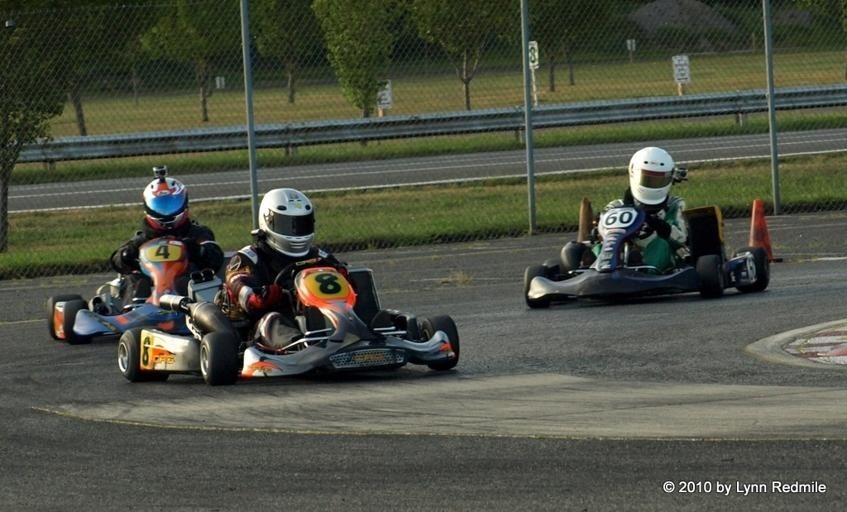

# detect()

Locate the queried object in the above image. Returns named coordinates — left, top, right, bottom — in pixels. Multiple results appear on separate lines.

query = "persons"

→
left=576, top=146, right=695, bottom=276
left=108, top=164, right=225, bottom=283
left=212, top=187, right=350, bottom=322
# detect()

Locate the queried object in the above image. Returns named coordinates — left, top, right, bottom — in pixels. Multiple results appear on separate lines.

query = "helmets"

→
left=142, top=177, right=192, bottom=232
left=627, top=146, right=688, bottom=206
left=250, top=187, right=316, bottom=258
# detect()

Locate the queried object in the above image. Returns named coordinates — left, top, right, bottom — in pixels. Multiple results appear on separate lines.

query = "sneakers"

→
left=581, top=248, right=597, bottom=269
left=628, top=250, right=644, bottom=271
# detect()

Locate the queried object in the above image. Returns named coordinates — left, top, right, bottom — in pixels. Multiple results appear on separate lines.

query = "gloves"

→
left=644, top=213, right=671, bottom=240
left=121, top=247, right=139, bottom=268
left=182, top=239, right=202, bottom=264
left=246, top=284, right=282, bottom=313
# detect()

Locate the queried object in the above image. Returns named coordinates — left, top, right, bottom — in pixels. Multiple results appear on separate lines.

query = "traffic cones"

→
left=575, top=196, right=594, bottom=246
left=738, top=198, right=783, bottom=263
